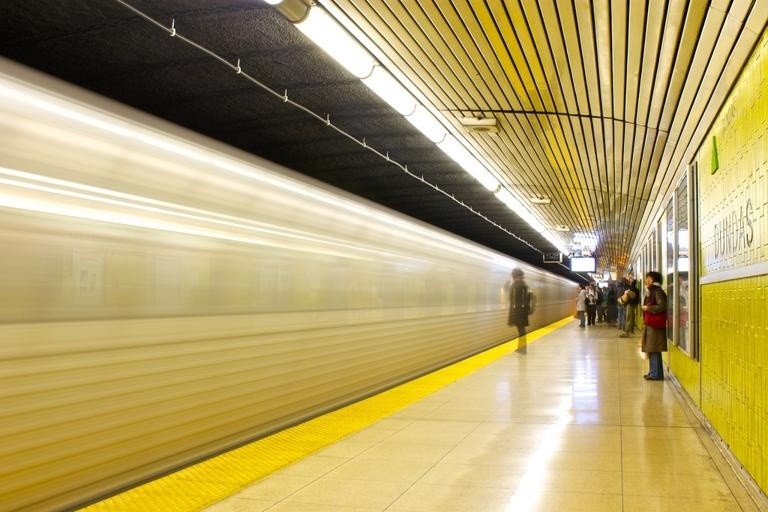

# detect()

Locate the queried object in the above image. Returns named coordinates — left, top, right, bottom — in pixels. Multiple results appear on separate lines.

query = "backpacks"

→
left=585, top=297, right=590, bottom=306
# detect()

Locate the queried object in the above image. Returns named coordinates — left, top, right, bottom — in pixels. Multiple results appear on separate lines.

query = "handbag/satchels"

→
left=527, top=291, right=537, bottom=315
left=644, top=303, right=667, bottom=328
left=617, top=290, right=636, bottom=305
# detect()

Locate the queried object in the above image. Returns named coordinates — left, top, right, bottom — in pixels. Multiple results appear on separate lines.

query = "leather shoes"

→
left=644, top=375, right=655, bottom=380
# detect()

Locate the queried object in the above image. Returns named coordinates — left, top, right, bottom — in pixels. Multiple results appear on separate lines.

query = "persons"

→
left=575, top=279, right=640, bottom=337
left=641, top=272, right=667, bottom=380
left=508, top=268, right=528, bottom=354
left=668, top=274, right=688, bottom=339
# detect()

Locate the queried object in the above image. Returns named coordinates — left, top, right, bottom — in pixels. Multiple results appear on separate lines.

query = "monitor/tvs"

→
left=570, top=256, right=597, bottom=273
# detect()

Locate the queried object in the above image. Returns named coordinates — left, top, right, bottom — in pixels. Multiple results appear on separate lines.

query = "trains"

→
left=1, top=53, right=582, bottom=511
